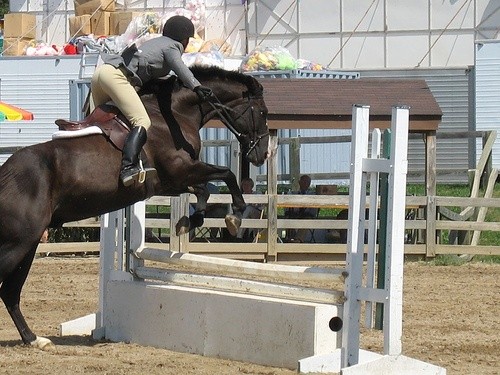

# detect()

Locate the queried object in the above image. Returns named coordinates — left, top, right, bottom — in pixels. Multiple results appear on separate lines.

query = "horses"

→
left=0, top=65, right=269, bottom=351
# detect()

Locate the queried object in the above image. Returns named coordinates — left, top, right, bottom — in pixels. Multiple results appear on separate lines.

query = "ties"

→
left=298, top=190, right=305, bottom=216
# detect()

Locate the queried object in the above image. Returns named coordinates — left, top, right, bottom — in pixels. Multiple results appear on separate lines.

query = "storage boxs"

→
left=110, top=11, right=144, bottom=34
left=69, top=14, right=91, bottom=36
left=4, top=12, right=36, bottom=39
left=74, top=0, right=115, bottom=15
left=91, top=11, right=110, bottom=36
left=2, top=38, right=31, bottom=56
left=315, top=184, right=339, bottom=195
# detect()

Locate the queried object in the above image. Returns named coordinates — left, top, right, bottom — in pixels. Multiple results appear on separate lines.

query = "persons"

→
left=65, top=39, right=86, bottom=55
left=92, top=15, right=214, bottom=187
left=282, top=175, right=321, bottom=243
left=230, top=176, right=263, bottom=242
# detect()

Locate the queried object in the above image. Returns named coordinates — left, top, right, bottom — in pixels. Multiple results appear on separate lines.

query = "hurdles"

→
left=58, top=103, right=448, bottom=375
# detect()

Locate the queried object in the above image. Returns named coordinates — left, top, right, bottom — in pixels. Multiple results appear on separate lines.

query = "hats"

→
left=163, top=16, right=196, bottom=42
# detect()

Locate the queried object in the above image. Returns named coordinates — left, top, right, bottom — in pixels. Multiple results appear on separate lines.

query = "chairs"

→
left=192, top=208, right=319, bottom=243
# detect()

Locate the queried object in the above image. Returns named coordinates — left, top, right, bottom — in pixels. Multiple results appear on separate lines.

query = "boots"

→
left=119, top=125, right=147, bottom=201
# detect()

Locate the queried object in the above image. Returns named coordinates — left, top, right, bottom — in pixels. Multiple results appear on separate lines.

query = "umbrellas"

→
left=0, top=102, right=34, bottom=121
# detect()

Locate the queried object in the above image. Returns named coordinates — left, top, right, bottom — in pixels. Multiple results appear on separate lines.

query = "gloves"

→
left=193, top=86, right=213, bottom=101
